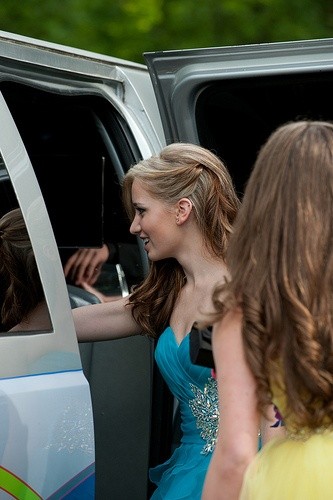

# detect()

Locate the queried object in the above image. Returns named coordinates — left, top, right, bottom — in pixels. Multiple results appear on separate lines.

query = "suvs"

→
left=0, top=27, right=333, bottom=498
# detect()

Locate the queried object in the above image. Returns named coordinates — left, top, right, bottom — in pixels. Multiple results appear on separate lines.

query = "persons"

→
left=70, top=142, right=286, bottom=500
left=64, top=244, right=130, bottom=303
left=0, top=208, right=53, bottom=333
left=194, top=121, right=333, bottom=500
